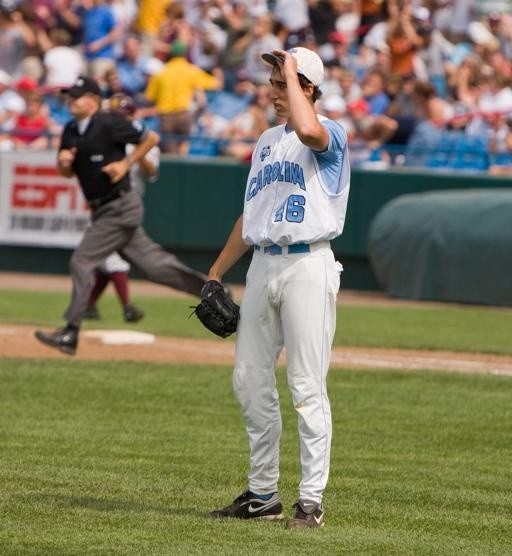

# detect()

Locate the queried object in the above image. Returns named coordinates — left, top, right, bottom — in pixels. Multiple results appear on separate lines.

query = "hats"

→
left=261, top=47, right=325, bottom=87
left=60, top=76, right=101, bottom=99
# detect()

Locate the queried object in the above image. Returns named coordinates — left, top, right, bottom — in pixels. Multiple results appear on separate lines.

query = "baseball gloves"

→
left=187, top=280, right=241, bottom=339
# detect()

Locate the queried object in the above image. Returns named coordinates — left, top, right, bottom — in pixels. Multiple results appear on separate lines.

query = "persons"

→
left=0, top=0, right=512, bottom=177
left=195, top=46, right=351, bottom=530
left=82, top=93, right=162, bottom=322
left=35, top=75, right=231, bottom=356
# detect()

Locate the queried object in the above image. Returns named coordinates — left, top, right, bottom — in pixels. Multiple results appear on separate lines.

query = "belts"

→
left=256, top=243, right=309, bottom=254
left=87, top=183, right=131, bottom=209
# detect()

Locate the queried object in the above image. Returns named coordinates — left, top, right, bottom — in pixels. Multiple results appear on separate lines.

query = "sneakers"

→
left=86, top=305, right=100, bottom=319
left=35, top=325, right=78, bottom=355
left=204, top=491, right=283, bottom=521
left=125, top=307, right=143, bottom=323
left=286, top=499, right=325, bottom=529
